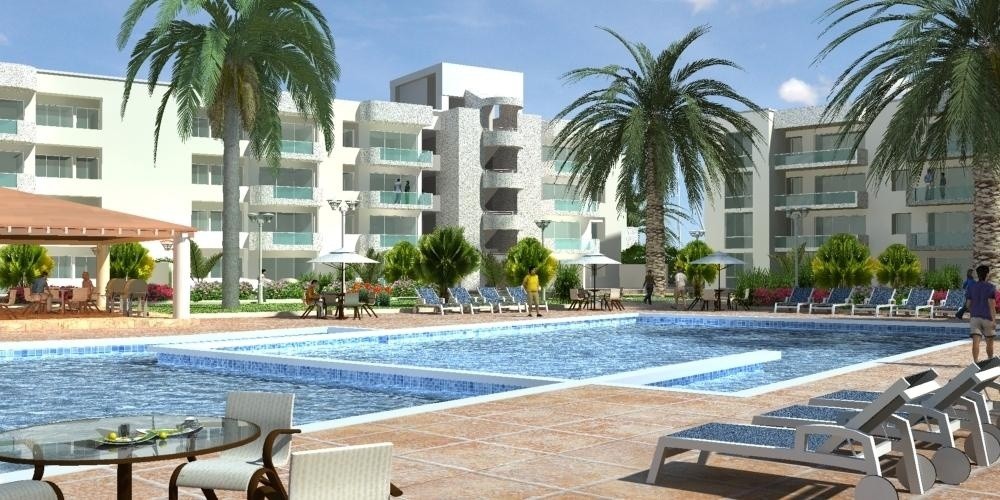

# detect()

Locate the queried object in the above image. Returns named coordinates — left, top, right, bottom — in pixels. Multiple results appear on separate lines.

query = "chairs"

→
left=568, top=287, right=625, bottom=311
left=169, top=390, right=302, bottom=500
left=645, top=369, right=942, bottom=500
left=411, top=287, right=548, bottom=316
left=0, top=286, right=101, bottom=314
left=684, top=284, right=968, bottom=320
left=300, top=291, right=379, bottom=319
left=255, top=441, right=404, bottom=499
left=753, top=356, right=1000, bottom=485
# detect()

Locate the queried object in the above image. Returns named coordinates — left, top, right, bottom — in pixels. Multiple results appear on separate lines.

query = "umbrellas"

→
left=306, top=248, right=381, bottom=320
left=561, top=249, right=623, bottom=310
left=688, top=252, right=748, bottom=311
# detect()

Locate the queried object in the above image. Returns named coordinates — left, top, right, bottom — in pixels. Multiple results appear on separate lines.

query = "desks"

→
left=0, top=414, right=261, bottom=500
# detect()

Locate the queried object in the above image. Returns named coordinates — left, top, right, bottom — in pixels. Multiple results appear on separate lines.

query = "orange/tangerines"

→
left=159, top=431, right=168, bottom=440
left=107, top=432, right=117, bottom=441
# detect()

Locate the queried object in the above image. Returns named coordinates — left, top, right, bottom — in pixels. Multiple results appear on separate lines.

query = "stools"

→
left=0, top=441, right=65, bottom=500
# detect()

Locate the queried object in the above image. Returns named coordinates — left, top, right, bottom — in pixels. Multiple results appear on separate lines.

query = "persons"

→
left=965, top=265, right=998, bottom=365
left=306, top=280, right=327, bottom=318
left=939, top=172, right=945, bottom=200
left=257, top=269, right=267, bottom=303
left=522, top=265, right=543, bottom=317
left=404, top=181, right=410, bottom=204
left=393, top=179, right=403, bottom=203
left=924, top=169, right=932, bottom=201
left=643, top=270, right=656, bottom=306
left=955, top=270, right=975, bottom=319
left=80, top=271, right=93, bottom=303
left=670, top=266, right=687, bottom=309
left=32, top=272, right=53, bottom=313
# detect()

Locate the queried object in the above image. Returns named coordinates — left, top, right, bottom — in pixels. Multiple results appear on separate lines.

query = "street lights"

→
left=248, top=211, right=275, bottom=305
left=328, top=198, right=360, bottom=296
left=533, top=218, right=552, bottom=303
left=776, top=206, right=812, bottom=290
left=690, top=229, right=706, bottom=248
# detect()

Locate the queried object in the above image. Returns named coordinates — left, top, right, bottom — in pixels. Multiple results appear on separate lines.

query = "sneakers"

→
left=537, top=313, right=542, bottom=317
